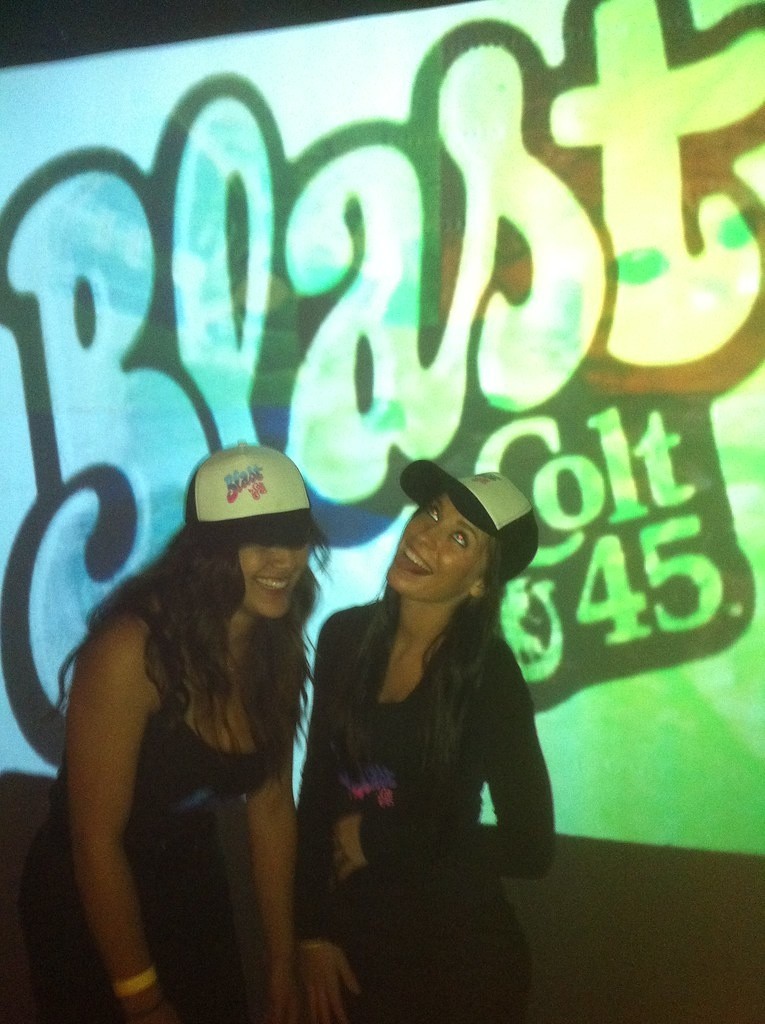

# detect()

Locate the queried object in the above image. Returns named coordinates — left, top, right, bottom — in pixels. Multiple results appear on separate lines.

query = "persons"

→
left=19, top=441, right=330, bottom=1024
left=293, top=460, right=555, bottom=1024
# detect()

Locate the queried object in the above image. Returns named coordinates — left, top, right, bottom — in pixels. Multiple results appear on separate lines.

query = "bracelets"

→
left=111, top=965, right=157, bottom=997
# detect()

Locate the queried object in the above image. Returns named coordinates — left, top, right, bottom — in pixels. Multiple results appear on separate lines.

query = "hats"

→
left=186, top=442, right=311, bottom=543
left=400, top=460, right=539, bottom=584
